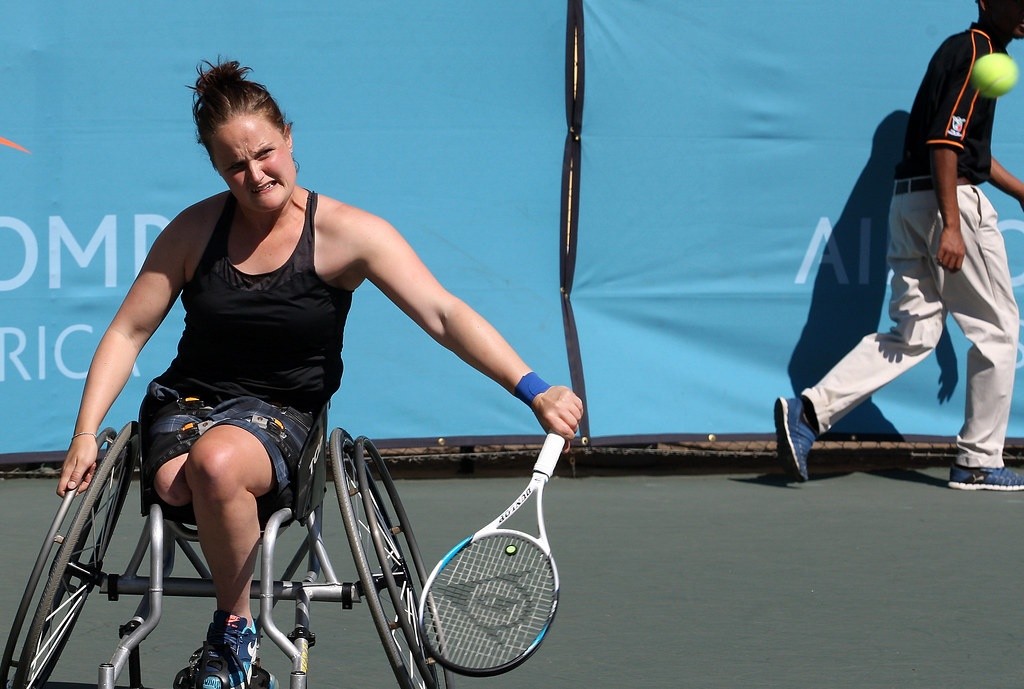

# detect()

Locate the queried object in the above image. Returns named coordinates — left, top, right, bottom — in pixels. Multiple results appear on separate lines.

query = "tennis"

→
left=970, top=53, right=1019, bottom=100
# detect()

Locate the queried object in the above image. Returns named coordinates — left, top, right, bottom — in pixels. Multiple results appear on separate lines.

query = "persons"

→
left=54, top=54, right=584, bottom=689
left=774, top=0, right=1024, bottom=491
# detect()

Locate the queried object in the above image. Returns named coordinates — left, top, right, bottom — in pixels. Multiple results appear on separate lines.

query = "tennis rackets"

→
left=414, top=427, right=568, bottom=679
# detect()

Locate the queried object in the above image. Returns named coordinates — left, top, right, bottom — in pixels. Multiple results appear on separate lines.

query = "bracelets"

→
left=72, top=431, right=98, bottom=440
left=511, top=371, right=551, bottom=407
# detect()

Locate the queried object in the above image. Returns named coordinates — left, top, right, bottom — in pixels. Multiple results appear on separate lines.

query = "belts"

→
left=895, top=172, right=971, bottom=194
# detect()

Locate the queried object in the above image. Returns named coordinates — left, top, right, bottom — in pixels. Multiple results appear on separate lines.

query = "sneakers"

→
left=773, top=396, right=817, bottom=482
left=948, top=462, right=1024, bottom=490
left=189, top=610, right=260, bottom=689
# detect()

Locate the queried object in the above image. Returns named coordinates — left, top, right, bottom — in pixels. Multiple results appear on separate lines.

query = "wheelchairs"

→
left=0, top=422, right=451, bottom=689
